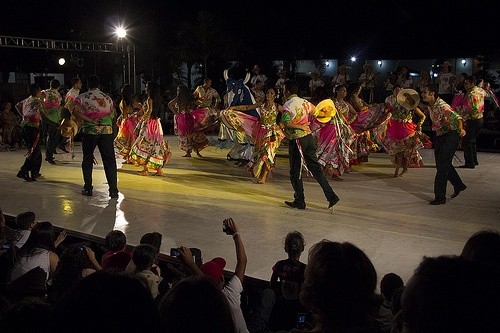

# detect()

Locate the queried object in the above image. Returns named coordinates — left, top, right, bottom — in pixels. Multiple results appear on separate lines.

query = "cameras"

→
left=222, top=221, right=233, bottom=235
left=170, top=248, right=180, bottom=256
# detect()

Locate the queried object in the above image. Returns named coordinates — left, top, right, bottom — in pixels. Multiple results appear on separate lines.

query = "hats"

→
left=200, top=258, right=226, bottom=285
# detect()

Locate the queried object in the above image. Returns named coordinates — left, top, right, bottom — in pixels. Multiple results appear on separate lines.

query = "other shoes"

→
left=182, top=153, right=191, bottom=157
left=153, top=172, right=163, bottom=176
left=196, top=153, right=202, bottom=157
left=238, top=160, right=265, bottom=184
left=138, top=170, right=149, bottom=176
left=333, top=175, right=343, bottom=180
left=122, top=160, right=132, bottom=164
left=394, top=171, right=401, bottom=177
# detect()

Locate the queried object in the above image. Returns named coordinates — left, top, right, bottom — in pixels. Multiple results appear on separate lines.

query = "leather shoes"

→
left=31, top=173, right=41, bottom=179
left=458, top=165, right=475, bottom=169
left=58, top=145, right=69, bottom=153
left=110, top=192, right=118, bottom=199
left=451, top=183, right=467, bottom=198
left=475, top=162, right=479, bottom=165
left=326, top=193, right=340, bottom=208
left=82, top=189, right=92, bottom=196
left=17, top=170, right=33, bottom=182
left=429, top=197, right=446, bottom=205
left=285, top=200, right=306, bottom=209
left=48, top=159, right=56, bottom=164
left=54, top=147, right=57, bottom=154
left=45, top=157, right=55, bottom=160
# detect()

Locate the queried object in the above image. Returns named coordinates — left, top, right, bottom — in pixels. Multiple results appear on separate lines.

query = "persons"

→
left=59, top=78, right=83, bottom=152
left=0, top=85, right=30, bottom=151
left=280, top=84, right=340, bottom=211
left=71, top=73, right=119, bottom=200
left=41, top=79, right=62, bottom=165
left=17, top=83, right=46, bottom=181
left=132, top=81, right=166, bottom=176
left=418, top=83, right=467, bottom=205
left=162, top=60, right=500, bottom=185
left=0, top=207, right=499, bottom=332
left=116, top=84, right=142, bottom=165
